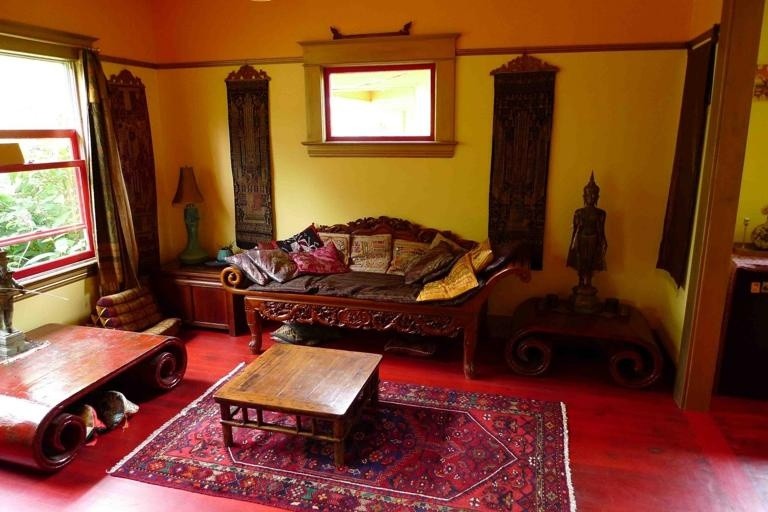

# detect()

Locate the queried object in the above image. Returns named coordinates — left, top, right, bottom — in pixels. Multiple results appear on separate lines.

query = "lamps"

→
left=172, top=163, right=210, bottom=266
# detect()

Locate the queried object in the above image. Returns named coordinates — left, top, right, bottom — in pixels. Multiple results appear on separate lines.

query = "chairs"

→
left=94, top=286, right=182, bottom=336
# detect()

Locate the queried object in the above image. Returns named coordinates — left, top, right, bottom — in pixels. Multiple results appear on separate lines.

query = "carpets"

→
left=107, top=358, right=586, bottom=512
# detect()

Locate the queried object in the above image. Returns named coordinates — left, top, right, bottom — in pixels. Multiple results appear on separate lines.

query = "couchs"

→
left=219, top=213, right=533, bottom=381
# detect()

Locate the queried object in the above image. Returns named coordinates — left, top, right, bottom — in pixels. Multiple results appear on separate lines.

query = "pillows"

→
left=351, top=233, right=497, bottom=305
left=222, top=223, right=348, bottom=287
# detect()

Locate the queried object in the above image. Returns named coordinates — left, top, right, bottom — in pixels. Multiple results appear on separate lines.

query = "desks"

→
left=2, top=322, right=190, bottom=473
left=713, top=244, right=767, bottom=404
left=505, top=298, right=663, bottom=388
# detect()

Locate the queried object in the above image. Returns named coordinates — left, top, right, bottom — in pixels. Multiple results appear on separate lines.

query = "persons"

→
left=0, top=250, right=24, bottom=333
left=566, top=169, right=608, bottom=287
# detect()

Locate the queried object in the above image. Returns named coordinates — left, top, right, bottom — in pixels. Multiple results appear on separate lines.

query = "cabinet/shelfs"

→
left=154, top=255, right=244, bottom=336
